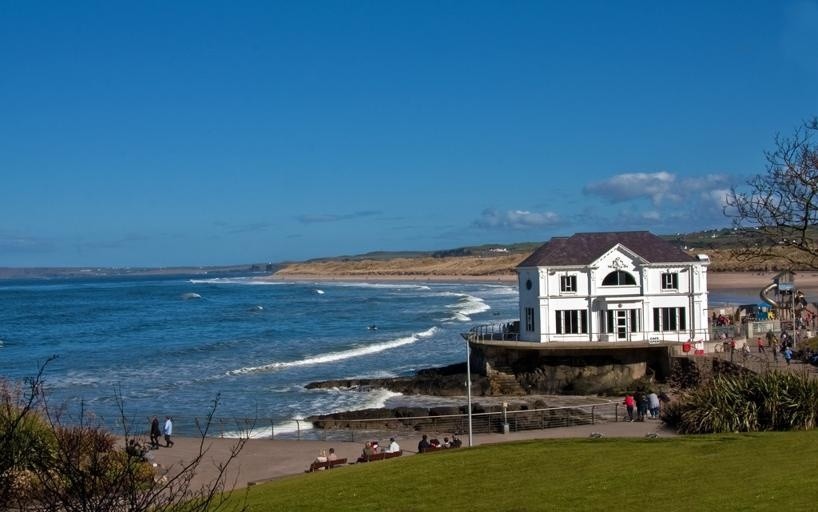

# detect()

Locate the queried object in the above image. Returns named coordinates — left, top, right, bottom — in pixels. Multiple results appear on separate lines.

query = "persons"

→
left=164, top=415, right=174, bottom=447
left=314, top=448, right=327, bottom=470
left=646, top=390, right=660, bottom=419
left=633, top=391, right=647, bottom=422
left=712, top=310, right=818, bottom=365
left=327, top=447, right=337, bottom=462
left=385, top=437, right=400, bottom=453
left=150, top=415, right=161, bottom=449
left=623, top=393, right=634, bottom=422
left=418, top=434, right=462, bottom=453
left=362, top=440, right=381, bottom=456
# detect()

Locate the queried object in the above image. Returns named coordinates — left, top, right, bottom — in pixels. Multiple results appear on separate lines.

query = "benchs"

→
left=305, top=449, right=402, bottom=474
